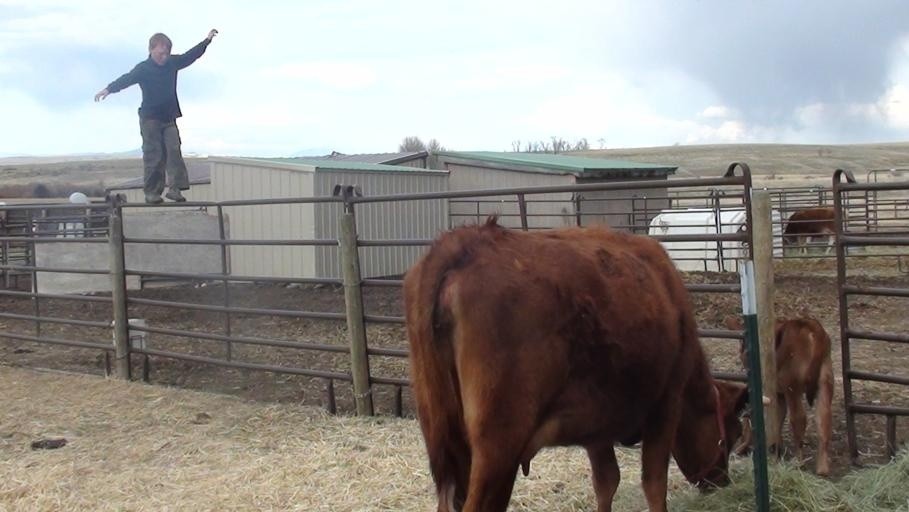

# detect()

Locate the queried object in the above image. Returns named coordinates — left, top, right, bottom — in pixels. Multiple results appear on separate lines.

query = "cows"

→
left=781, top=203, right=848, bottom=255
left=399, top=211, right=771, bottom=511
left=724, top=310, right=834, bottom=477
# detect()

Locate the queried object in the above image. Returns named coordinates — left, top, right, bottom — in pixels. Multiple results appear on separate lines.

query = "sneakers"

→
left=144, top=193, right=164, bottom=203
left=164, top=187, right=187, bottom=201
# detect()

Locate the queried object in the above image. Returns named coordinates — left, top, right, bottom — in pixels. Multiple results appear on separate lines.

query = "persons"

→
left=93, top=27, right=219, bottom=204
